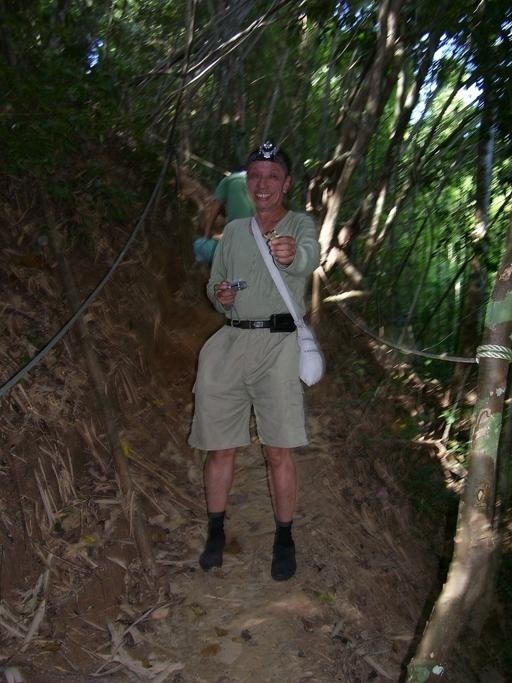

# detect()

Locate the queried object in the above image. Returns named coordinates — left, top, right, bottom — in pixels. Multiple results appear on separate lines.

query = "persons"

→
left=188, top=139, right=322, bottom=582
left=203, top=166, right=254, bottom=241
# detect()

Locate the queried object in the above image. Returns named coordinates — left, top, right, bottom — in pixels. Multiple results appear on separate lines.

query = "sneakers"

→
left=199, top=534, right=224, bottom=574
left=270, top=536, right=296, bottom=582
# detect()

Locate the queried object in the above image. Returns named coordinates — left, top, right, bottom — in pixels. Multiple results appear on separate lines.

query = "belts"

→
left=222, top=318, right=272, bottom=329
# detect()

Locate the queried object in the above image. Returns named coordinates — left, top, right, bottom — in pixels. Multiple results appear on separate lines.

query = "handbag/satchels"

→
left=295, top=322, right=328, bottom=388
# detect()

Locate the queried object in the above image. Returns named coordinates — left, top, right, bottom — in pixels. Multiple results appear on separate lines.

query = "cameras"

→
left=227, top=279, right=248, bottom=292
